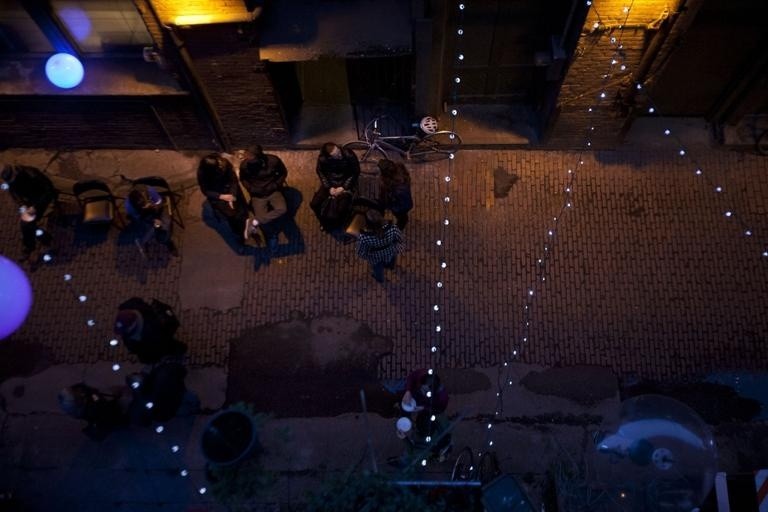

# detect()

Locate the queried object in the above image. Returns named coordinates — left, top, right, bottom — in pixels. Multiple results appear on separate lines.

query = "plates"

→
left=20, top=211, right=36, bottom=222
left=402, top=397, right=417, bottom=412
left=395, top=417, right=412, bottom=434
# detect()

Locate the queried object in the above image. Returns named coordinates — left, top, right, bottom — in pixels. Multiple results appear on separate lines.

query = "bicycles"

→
left=342, top=115, right=462, bottom=176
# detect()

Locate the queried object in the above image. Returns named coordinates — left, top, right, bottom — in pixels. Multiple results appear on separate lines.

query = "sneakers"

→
left=17, top=253, right=30, bottom=262
left=244, top=218, right=256, bottom=240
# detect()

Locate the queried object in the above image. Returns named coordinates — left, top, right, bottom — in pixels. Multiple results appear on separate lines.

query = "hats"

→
left=114, top=309, right=139, bottom=338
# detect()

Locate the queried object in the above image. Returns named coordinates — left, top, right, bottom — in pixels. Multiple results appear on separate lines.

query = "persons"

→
left=117, top=365, right=185, bottom=429
left=194, top=153, right=271, bottom=269
left=112, top=297, right=190, bottom=371
left=374, top=157, right=416, bottom=233
left=238, top=143, right=290, bottom=254
left=309, top=141, right=361, bottom=240
left=354, top=209, right=404, bottom=283
left=123, top=181, right=180, bottom=259
left=387, top=368, right=452, bottom=440
left=0, top=160, right=58, bottom=264
left=55, top=382, right=131, bottom=431
